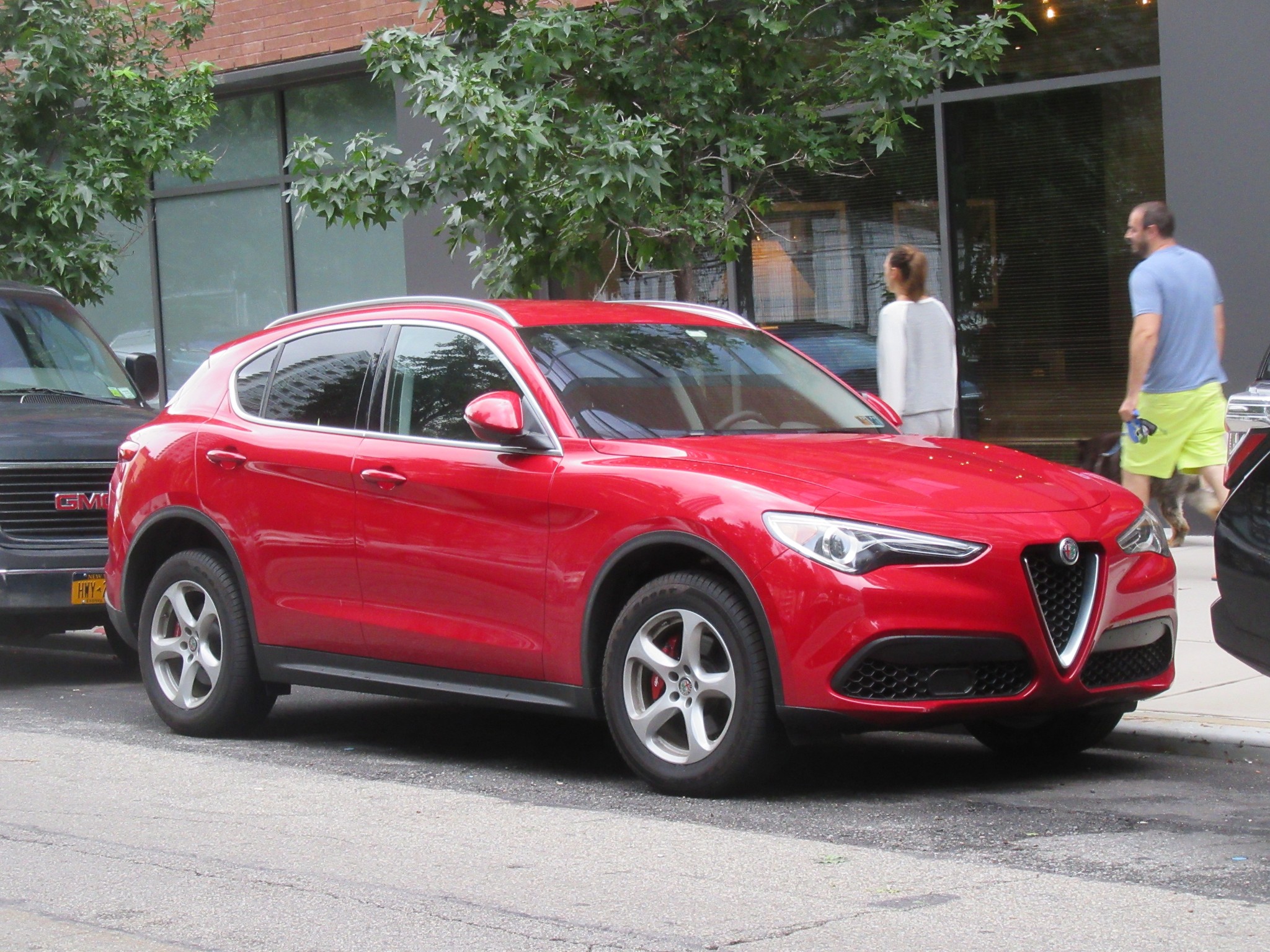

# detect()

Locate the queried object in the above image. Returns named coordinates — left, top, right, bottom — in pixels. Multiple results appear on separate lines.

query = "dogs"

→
left=1075, top=433, right=1220, bottom=546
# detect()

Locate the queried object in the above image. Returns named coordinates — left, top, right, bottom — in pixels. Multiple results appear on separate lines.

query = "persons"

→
left=1117, top=200, right=1231, bottom=582
left=876, top=243, right=957, bottom=438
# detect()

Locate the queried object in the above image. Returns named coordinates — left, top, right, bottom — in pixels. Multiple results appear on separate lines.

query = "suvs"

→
left=0, top=280, right=165, bottom=647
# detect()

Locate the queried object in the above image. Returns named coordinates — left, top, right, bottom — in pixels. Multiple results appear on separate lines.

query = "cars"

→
left=1209, top=342, right=1270, bottom=678
left=747, top=318, right=991, bottom=440
left=99, top=290, right=1182, bottom=800
